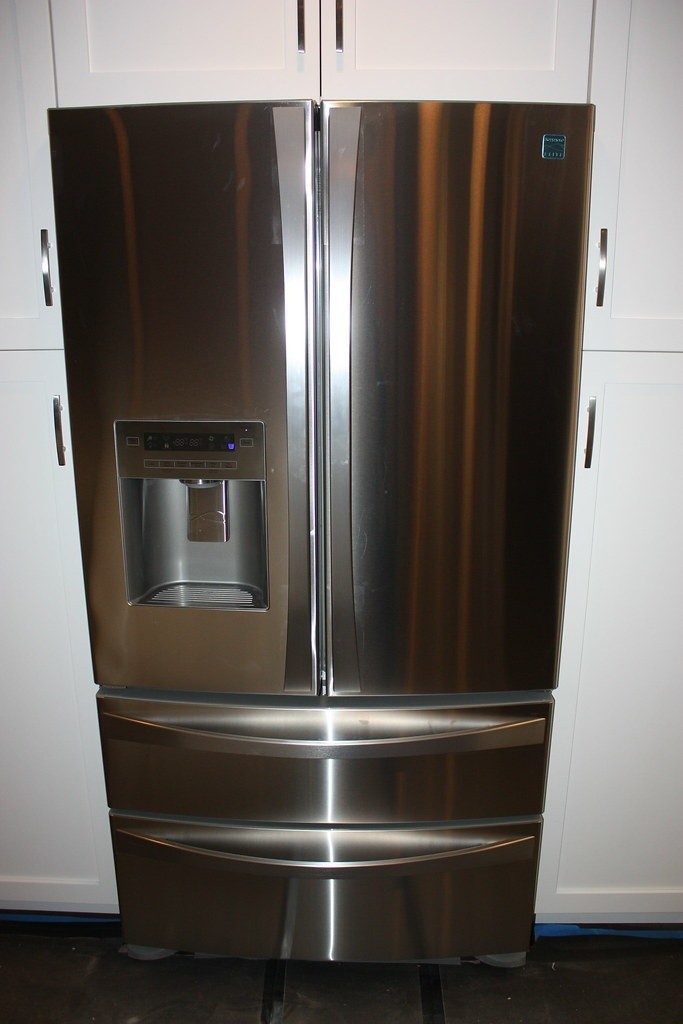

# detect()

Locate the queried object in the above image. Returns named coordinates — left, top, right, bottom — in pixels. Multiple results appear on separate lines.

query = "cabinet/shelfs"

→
left=0, top=0, right=123, bottom=917
left=531, top=0, right=683, bottom=936
left=49, top=2, right=592, bottom=101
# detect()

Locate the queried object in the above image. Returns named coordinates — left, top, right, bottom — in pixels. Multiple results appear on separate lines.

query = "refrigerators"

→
left=42, top=93, right=600, bottom=974
